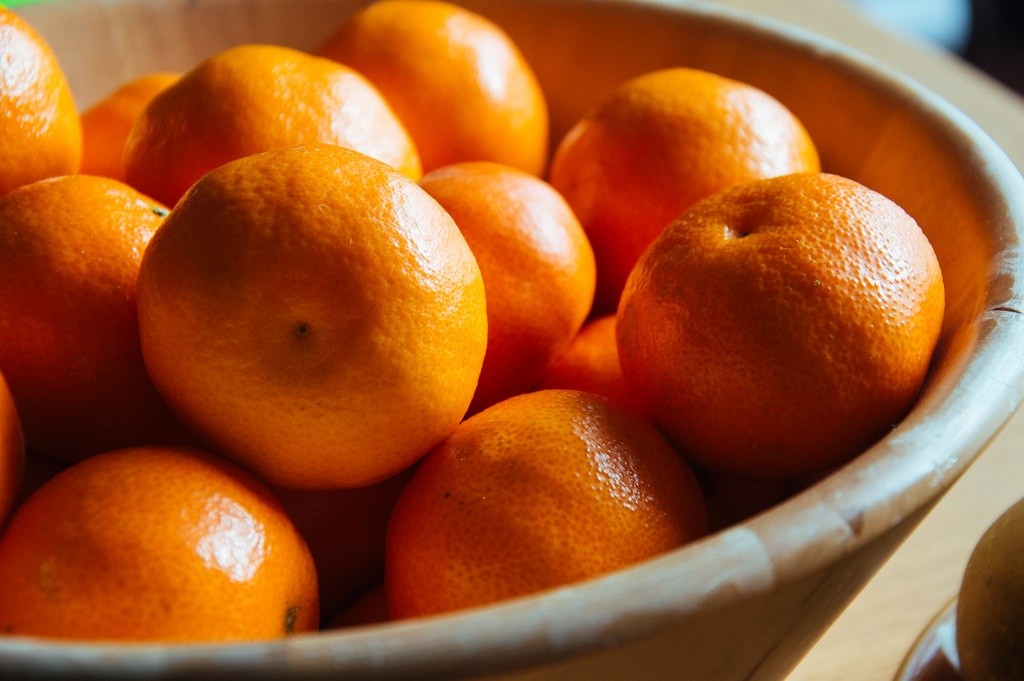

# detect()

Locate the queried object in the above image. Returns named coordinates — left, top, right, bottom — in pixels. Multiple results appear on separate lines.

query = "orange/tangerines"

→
left=0, top=0, right=945, bottom=644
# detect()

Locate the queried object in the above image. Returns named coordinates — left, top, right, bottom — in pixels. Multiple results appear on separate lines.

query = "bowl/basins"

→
left=0, top=1, right=1024, bottom=681
